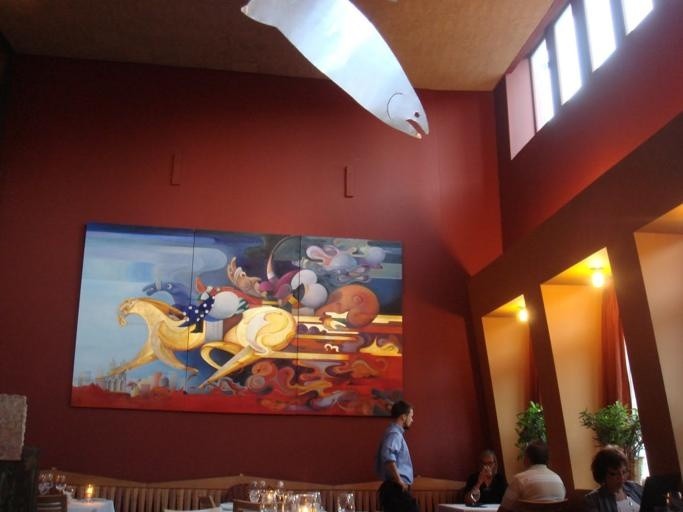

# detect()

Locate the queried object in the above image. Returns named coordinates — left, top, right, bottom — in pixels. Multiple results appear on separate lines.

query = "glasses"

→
left=480, top=461, right=496, bottom=466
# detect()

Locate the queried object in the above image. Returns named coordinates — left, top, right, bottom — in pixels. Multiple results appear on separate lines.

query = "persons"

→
left=581, top=448, right=644, bottom=512
left=376, top=400, right=415, bottom=512
left=464, top=450, right=507, bottom=505
left=501, top=441, right=566, bottom=508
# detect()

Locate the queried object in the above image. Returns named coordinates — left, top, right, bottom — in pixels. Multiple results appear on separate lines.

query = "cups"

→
left=338, top=492, right=355, bottom=512
left=249, top=481, right=324, bottom=511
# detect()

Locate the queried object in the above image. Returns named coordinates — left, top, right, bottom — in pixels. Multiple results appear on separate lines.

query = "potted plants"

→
left=578, top=401, right=643, bottom=482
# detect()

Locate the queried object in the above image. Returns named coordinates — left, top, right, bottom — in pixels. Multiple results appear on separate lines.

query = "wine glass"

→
left=470, top=488, right=480, bottom=507
left=38, top=471, right=75, bottom=495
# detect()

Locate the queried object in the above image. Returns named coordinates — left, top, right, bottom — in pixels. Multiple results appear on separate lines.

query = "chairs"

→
left=197, top=494, right=215, bottom=508
left=35, top=493, right=68, bottom=512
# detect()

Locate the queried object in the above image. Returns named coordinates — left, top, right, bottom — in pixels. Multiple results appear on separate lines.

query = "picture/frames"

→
left=66, top=218, right=406, bottom=418
